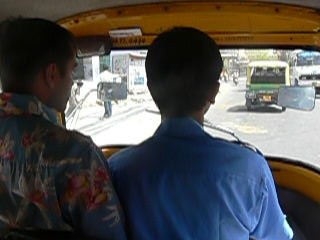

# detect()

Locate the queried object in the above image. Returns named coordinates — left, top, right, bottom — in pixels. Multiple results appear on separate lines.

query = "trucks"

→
left=290, top=50, right=320, bottom=96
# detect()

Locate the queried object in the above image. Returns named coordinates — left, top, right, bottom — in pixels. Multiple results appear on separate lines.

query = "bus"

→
left=246, top=60, right=290, bottom=112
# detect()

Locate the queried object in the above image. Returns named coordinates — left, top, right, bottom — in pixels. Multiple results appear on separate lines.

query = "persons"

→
left=255, top=67, right=284, bottom=77
left=0, top=17, right=126, bottom=240
left=65, top=81, right=83, bottom=117
left=220, top=68, right=239, bottom=86
left=97, top=64, right=113, bottom=119
left=107, top=26, right=294, bottom=240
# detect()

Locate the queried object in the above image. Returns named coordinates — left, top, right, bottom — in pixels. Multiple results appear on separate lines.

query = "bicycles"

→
left=233, top=76, right=238, bottom=85
